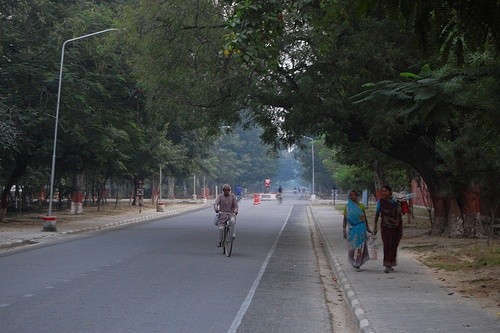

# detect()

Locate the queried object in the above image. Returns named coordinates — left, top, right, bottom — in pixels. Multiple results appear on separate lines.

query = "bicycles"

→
left=217, top=211, right=237, bottom=257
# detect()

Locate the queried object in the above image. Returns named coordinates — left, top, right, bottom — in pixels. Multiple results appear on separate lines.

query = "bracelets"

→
left=343, top=231, right=346, bottom=232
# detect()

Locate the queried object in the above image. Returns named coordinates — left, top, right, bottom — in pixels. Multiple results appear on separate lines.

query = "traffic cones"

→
left=257, top=194, right=261, bottom=204
left=253, top=195, right=258, bottom=205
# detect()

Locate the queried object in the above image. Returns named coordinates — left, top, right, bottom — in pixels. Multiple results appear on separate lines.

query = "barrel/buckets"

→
left=369, top=244, right=378, bottom=260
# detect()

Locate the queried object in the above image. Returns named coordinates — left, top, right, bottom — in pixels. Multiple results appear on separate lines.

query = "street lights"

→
left=47, top=28, right=128, bottom=217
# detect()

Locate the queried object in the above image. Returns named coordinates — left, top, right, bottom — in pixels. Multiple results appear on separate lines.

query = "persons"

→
left=343, top=190, right=373, bottom=272
left=294, top=186, right=306, bottom=194
left=214, top=184, right=239, bottom=245
left=278, top=185, right=283, bottom=198
left=264, top=177, right=271, bottom=194
left=374, top=186, right=412, bottom=273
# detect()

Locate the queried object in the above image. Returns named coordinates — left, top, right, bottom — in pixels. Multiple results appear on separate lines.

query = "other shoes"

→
left=233, top=234, right=235, bottom=238
left=384, top=267, right=394, bottom=273
left=355, top=267, right=361, bottom=272
left=219, top=240, right=222, bottom=243
